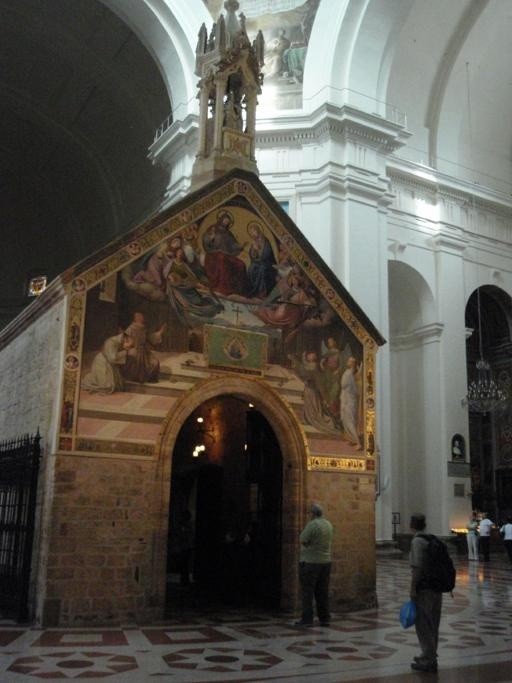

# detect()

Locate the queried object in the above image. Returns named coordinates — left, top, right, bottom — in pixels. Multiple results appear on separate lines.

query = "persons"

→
left=132, top=210, right=333, bottom=346
left=78, top=331, right=136, bottom=395
left=293, top=503, right=334, bottom=628
left=269, top=26, right=295, bottom=83
left=121, top=308, right=168, bottom=384
left=285, top=334, right=364, bottom=452
left=478, top=512, right=495, bottom=561
left=407, top=510, right=442, bottom=672
left=466, top=513, right=478, bottom=560
left=498, top=517, right=512, bottom=562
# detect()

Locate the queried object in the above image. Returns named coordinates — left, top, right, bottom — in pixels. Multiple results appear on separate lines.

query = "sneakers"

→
left=411, top=655, right=439, bottom=674
left=319, top=620, right=331, bottom=627
left=294, top=619, right=314, bottom=628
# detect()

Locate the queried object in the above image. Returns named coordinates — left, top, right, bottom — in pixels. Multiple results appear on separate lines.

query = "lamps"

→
left=461, top=61, right=506, bottom=416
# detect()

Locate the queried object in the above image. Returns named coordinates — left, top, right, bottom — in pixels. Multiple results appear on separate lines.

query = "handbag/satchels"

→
left=399, top=600, right=416, bottom=629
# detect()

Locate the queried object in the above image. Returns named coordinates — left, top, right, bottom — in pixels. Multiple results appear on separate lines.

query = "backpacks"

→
left=414, top=533, right=456, bottom=594
